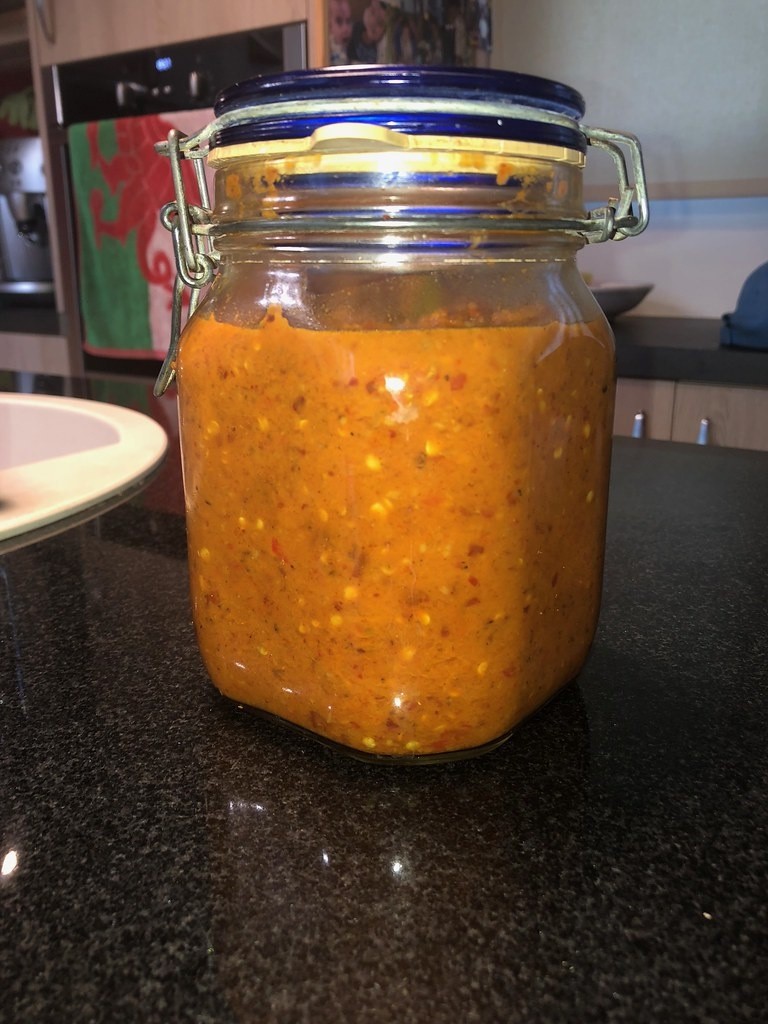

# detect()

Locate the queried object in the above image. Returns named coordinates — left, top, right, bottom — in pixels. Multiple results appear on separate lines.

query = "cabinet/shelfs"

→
left=610, top=379, right=768, bottom=455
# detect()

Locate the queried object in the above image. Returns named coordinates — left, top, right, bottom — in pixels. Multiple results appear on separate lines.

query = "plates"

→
left=0, top=389, right=168, bottom=557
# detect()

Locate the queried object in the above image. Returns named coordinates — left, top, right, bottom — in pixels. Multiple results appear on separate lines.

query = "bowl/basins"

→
left=588, top=279, right=653, bottom=325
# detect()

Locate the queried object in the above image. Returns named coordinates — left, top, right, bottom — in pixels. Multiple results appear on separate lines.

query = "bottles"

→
left=155, top=65, right=649, bottom=764
